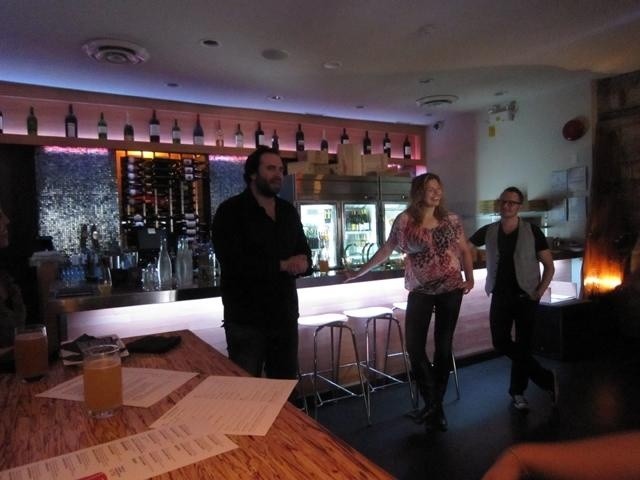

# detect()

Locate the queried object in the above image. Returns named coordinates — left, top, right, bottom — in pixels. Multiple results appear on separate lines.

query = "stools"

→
left=292, top=302, right=461, bottom=427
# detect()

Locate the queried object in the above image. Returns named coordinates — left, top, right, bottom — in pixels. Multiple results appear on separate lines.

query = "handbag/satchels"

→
left=126, top=336, right=181, bottom=353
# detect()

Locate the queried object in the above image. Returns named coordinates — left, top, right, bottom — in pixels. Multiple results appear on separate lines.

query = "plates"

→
left=60, top=331, right=129, bottom=367
left=568, top=247, right=584, bottom=252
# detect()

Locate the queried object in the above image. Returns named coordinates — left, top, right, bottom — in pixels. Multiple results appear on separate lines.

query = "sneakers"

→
left=548, top=369, right=559, bottom=403
left=512, top=395, right=528, bottom=410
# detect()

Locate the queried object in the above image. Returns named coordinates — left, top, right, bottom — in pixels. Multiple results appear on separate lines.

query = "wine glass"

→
left=29, top=249, right=65, bottom=313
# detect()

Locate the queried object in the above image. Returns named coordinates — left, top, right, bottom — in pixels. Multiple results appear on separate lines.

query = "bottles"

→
left=383, top=132, right=391, bottom=158
left=123, top=111, right=135, bottom=142
left=59, top=223, right=141, bottom=289
left=172, top=119, right=181, bottom=144
left=117, top=153, right=210, bottom=243
left=64, top=104, right=77, bottom=137
left=175, top=238, right=183, bottom=287
left=180, top=235, right=195, bottom=287
left=320, top=128, right=329, bottom=152
left=156, top=235, right=173, bottom=289
left=339, top=128, right=350, bottom=145
left=192, top=113, right=204, bottom=146
left=26, top=106, right=38, bottom=137
left=299, top=204, right=332, bottom=264
left=254, top=121, right=265, bottom=149
left=215, top=119, right=225, bottom=148
left=235, top=124, right=244, bottom=147
left=295, top=124, right=306, bottom=151
left=149, top=109, right=161, bottom=143
left=402, top=134, right=411, bottom=159
left=271, top=129, right=280, bottom=150
left=343, top=206, right=374, bottom=264
left=362, top=131, right=371, bottom=156
left=96, top=112, right=107, bottom=139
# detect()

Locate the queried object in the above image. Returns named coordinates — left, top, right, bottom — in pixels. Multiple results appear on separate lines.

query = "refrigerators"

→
left=275, top=170, right=380, bottom=276
left=378, top=175, right=422, bottom=266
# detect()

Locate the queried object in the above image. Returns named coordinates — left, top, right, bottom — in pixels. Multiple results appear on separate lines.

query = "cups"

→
left=553, top=238, right=560, bottom=249
left=80, top=343, right=125, bottom=421
left=198, top=253, right=218, bottom=282
left=142, top=268, right=159, bottom=292
left=98, top=267, right=112, bottom=294
left=12, top=321, right=50, bottom=383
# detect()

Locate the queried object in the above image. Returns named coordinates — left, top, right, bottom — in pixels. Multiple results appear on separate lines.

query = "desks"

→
left=0, top=329, right=398, bottom=480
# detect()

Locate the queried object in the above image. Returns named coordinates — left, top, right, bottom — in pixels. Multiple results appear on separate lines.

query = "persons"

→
left=467, top=186, right=555, bottom=412
left=1, top=209, right=27, bottom=374
left=211, top=147, right=313, bottom=402
left=343, top=172, right=473, bottom=428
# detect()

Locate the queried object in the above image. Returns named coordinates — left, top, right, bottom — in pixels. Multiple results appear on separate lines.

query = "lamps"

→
left=81, top=39, right=150, bottom=69
left=416, top=95, right=459, bottom=109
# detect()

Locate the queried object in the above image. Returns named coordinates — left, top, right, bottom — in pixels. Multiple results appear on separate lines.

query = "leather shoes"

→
left=416, top=404, right=447, bottom=431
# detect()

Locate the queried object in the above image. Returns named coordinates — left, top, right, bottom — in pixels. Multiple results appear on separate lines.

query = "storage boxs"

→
left=287, top=144, right=410, bottom=176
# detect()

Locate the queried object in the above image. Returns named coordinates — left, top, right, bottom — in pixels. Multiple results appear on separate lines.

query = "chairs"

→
left=537, top=279, right=607, bottom=361
